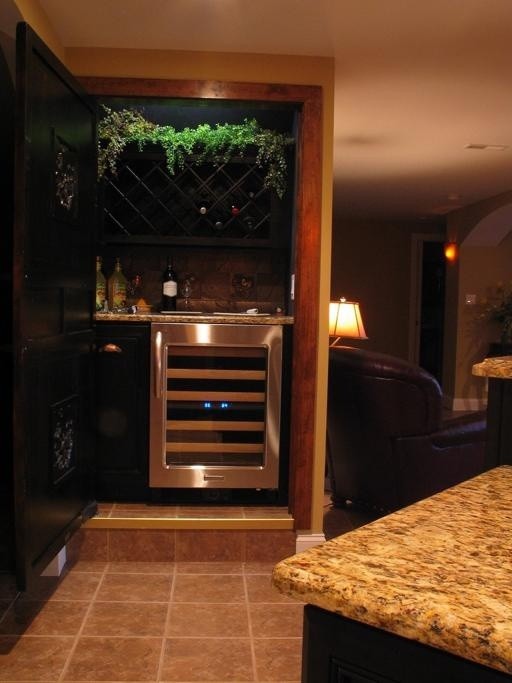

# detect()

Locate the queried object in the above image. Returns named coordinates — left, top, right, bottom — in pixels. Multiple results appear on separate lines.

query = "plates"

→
left=213, top=311, right=271, bottom=317
left=161, top=311, right=203, bottom=315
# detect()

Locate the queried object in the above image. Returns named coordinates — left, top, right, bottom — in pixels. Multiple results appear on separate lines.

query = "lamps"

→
left=328, top=294, right=368, bottom=352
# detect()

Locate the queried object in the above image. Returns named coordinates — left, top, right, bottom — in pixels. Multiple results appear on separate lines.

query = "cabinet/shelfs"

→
left=85, top=312, right=152, bottom=508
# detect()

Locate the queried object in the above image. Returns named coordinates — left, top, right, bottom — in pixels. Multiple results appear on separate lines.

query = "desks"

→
left=468, top=353, right=512, bottom=472
left=270, top=461, right=512, bottom=683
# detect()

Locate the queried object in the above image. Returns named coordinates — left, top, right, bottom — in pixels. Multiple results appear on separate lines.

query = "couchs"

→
left=322, top=340, right=492, bottom=523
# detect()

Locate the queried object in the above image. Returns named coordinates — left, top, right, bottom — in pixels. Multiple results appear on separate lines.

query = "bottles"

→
left=160, top=253, right=178, bottom=312
left=196, top=188, right=259, bottom=233
left=107, top=258, right=128, bottom=309
left=96, top=256, right=106, bottom=310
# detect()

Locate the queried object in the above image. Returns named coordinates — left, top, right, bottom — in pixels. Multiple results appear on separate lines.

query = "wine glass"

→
left=180, top=276, right=194, bottom=306
